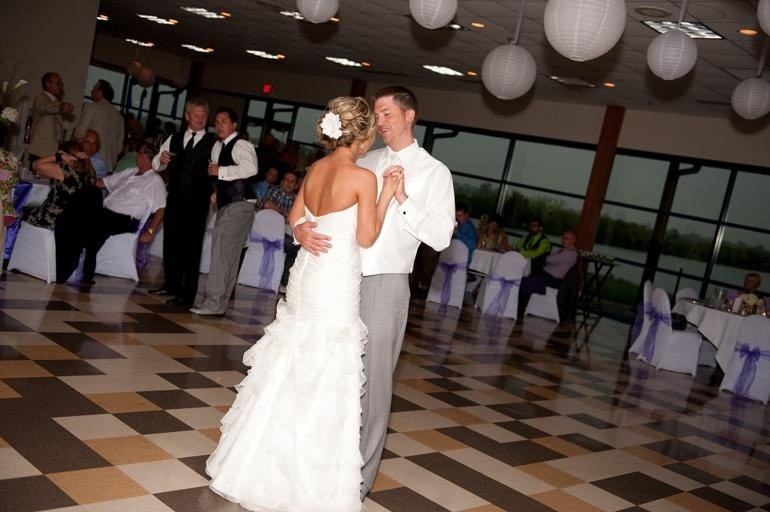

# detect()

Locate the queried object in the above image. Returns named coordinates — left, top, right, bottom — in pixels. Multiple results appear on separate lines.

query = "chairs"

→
left=236, top=207, right=287, bottom=296
left=675, top=287, right=699, bottom=299
left=638, top=288, right=701, bottom=377
left=523, top=285, right=562, bottom=324
left=624, top=281, right=652, bottom=354
left=86, top=205, right=152, bottom=285
left=144, top=214, right=216, bottom=272
left=480, top=248, right=528, bottom=321
left=425, top=239, right=469, bottom=310
left=6, top=220, right=58, bottom=287
left=718, top=314, right=770, bottom=405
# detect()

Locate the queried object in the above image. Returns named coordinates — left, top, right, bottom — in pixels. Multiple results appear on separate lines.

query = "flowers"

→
left=0, top=58, right=29, bottom=214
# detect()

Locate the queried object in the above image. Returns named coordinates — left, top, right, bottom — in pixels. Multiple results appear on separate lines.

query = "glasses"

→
left=188, top=111, right=209, bottom=120
left=136, top=150, right=148, bottom=155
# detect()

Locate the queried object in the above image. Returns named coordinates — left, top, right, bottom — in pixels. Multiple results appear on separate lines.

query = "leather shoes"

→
left=168, top=296, right=193, bottom=306
left=190, top=304, right=226, bottom=317
left=149, top=285, right=176, bottom=296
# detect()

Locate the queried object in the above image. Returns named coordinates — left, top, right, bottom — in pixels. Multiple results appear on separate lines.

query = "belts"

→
left=104, top=207, right=140, bottom=221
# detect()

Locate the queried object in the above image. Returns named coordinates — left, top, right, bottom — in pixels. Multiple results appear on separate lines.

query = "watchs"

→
left=148, top=226, right=157, bottom=236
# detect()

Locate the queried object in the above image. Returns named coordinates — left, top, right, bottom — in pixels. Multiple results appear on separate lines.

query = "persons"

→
left=78, top=79, right=126, bottom=170
left=123, top=112, right=177, bottom=157
left=189, top=110, right=258, bottom=317
left=28, top=73, right=74, bottom=173
left=725, top=273, right=766, bottom=312
left=253, top=131, right=328, bottom=192
left=57, top=143, right=166, bottom=286
left=516, top=218, right=553, bottom=277
left=477, top=213, right=509, bottom=253
left=255, top=166, right=280, bottom=210
left=259, top=173, right=299, bottom=296
left=420, top=206, right=477, bottom=296
left=152, top=99, right=216, bottom=310
left=20, top=141, right=99, bottom=228
left=516, top=230, right=579, bottom=317
left=206, top=96, right=402, bottom=511
left=290, top=88, right=456, bottom=501
left=76, top=129, right=107, bottom=177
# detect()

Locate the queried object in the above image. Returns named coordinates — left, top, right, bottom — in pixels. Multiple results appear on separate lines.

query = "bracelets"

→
left=55, top=152, right=63, bottom=162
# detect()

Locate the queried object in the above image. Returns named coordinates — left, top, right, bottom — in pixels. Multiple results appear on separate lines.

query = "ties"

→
left=185, top=132, right=197, bottom=152
left=220, top=142, right=226, bottom=153
left=527, top=238, right=535, bottom=249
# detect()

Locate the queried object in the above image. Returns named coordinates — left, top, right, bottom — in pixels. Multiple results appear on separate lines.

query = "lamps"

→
left=481, top=43, right=538, bottom=101
left=542, top=0, right=628, bottom=62
left=646, top=30, right=699, bottom=81
left=408, top=0, right=458, bottom=30
left=757, top=0, right=770, bottom=35
left=295, top=0, right=340, bottom=24
left=730, top=80, right=769, bottom=120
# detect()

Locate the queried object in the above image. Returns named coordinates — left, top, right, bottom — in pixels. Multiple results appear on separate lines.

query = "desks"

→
left=581, top=250, right=616, bottom=318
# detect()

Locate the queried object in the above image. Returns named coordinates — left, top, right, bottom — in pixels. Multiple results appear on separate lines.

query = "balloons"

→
left=138, top=67, right=157, bottom=87
left=126, top=58, right=142, bottom=76
